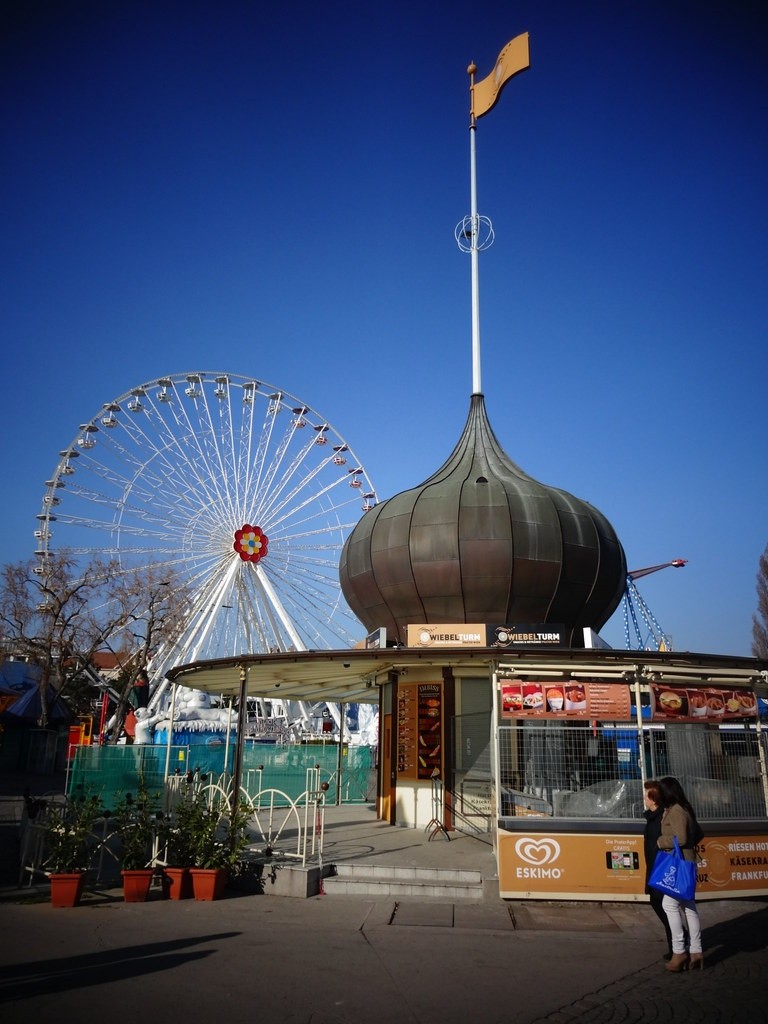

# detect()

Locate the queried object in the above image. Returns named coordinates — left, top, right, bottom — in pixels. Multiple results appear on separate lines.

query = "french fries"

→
left=693, top=696, right=706, bottom=708
left=709, top=698, right=723, bottom=710
left=739, top=696, right=755, bottom=708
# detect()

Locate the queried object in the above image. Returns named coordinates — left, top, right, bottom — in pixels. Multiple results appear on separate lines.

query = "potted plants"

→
left=41, top=788, right=107, bottom=908
left=109, top=787, right=160, bottom=903
left=190, top=795, right=257, bottom=901
left=160, top=784, right=207, bottom=901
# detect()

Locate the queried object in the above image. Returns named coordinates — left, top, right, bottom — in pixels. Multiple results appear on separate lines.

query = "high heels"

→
left=664, top=952, right=704, bottom=972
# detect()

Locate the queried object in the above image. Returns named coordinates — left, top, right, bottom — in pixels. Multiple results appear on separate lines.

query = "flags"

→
left=473, top=31, right=529, bottom=120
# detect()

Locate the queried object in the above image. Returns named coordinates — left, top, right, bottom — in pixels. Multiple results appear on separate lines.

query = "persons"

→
left=643, top=780, right=690, bottom=961
left=657, top=778, right=704, bottom=972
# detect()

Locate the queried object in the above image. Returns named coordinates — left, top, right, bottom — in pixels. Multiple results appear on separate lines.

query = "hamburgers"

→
left=660, top=692, right=682, bottom=711
left=503, top=693, right=521, bottom=710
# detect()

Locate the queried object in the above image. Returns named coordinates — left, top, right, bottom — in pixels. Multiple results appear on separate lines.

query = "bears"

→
left=133, top=707, right=166, bottom=771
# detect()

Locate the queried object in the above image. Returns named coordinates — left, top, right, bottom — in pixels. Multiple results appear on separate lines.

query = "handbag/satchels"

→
left=648, top=835, right=697, bottom=902
